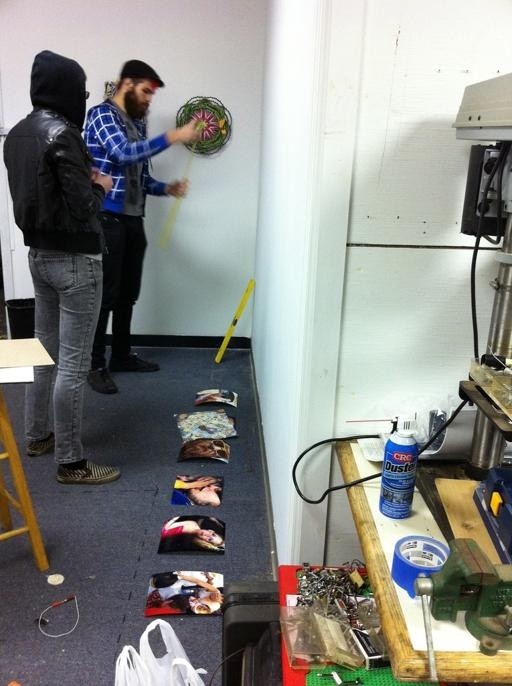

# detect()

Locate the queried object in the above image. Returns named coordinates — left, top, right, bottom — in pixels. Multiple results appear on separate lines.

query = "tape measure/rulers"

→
left=214, top=279, right=255, bottom=364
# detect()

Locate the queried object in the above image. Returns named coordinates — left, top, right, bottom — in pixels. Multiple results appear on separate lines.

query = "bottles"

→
left=378, top=416, right=420, bottom=520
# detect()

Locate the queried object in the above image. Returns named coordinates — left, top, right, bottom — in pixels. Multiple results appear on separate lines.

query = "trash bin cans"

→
left=4, top=298, right=35, bottom=339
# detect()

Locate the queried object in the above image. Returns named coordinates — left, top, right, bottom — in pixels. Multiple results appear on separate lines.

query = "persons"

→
left=142, top=571, right=223, bottom=616
left=2, top=49, right=124, bottom=485
left=178, top=437, right=231, bottom=462
left=82, top=59, right=203, bottom=395
left=159, top=514, right=225, bottom=554
left=170, top=472, right=222, bottom=507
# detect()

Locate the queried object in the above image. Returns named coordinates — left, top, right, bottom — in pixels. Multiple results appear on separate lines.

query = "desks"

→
left=324, top=406, right=512, bottom=683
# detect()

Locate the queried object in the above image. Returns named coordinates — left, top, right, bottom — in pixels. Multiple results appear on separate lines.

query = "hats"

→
left=121, top=60, right=164, bottom=87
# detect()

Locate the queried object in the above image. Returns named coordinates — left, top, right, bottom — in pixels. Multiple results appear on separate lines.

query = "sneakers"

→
left=122, top=352, right=159, bottom=372
left=87, top=368, right=117, bottom=394
left=56, top=460, right=120, bottom=485
left=25, top=432, right=54, bottom=457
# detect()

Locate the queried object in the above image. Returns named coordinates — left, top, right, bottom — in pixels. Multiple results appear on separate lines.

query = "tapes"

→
left=391, top=536, right=451, bottom=599
